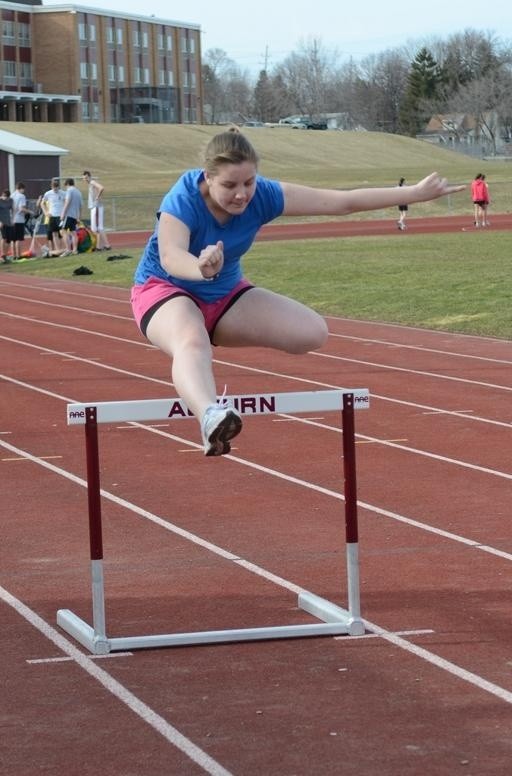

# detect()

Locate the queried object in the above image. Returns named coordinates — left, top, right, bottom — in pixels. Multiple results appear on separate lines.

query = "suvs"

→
left=296, top=116, right=327, bottom=130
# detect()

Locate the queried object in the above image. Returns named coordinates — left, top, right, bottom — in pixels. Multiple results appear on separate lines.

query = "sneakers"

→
left=0, top=245, right=112, bottom=263
left=199, top=384, right=242, bottom=457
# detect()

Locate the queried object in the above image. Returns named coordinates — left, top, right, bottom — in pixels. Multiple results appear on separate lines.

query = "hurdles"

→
left=56, top=389, right=370, bottom=656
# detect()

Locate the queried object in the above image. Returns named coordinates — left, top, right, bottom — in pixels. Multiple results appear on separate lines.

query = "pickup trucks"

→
left=263, top=117, right=307, bottom=129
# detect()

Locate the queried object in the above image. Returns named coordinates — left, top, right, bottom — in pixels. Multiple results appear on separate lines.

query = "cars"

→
left=242, top=121, right=265, bottom=129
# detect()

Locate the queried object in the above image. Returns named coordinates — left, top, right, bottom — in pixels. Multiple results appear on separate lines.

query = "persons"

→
left=471, top=173, right=486, bottom=229
left=128, top=126, right=468, bottom=457
left=1, top=171, right=112, bottom=263
left=482, top=175, right=491, bottom=226
left=397, top=176, right=408, bottom=230
